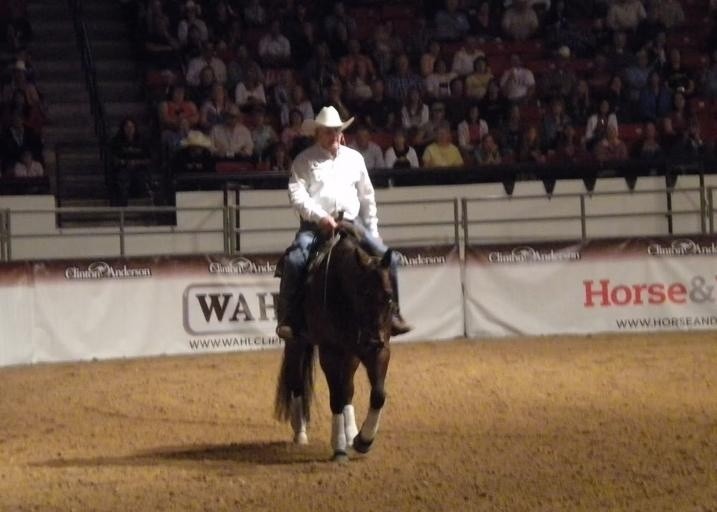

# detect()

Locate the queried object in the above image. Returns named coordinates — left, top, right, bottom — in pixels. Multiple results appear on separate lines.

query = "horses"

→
left=270, top=227, right=394, bottom=465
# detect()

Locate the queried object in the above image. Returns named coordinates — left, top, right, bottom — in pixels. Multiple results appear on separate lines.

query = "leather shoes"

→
left=278, top=325, right=294, bottom=337
left=391, top=314, right=409, bottom=333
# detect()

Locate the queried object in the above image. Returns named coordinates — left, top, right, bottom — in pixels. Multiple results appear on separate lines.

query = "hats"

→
left=302, top=106, right=355, bottom=136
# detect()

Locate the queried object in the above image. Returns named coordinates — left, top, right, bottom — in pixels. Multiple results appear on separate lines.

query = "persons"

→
left=276, top=106, right=412, bottom=337
left=1, top=1, right=717, bottom=227
